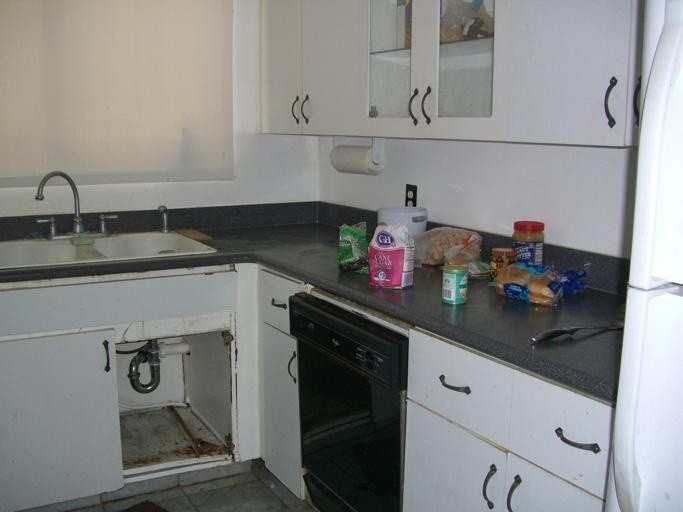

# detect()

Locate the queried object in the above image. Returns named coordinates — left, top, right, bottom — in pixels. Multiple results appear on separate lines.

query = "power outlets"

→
left=405, top=184, right=417, bottom=208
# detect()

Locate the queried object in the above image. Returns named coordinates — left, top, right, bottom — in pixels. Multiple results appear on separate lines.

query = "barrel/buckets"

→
left=377, top=206, right=428, bottom=238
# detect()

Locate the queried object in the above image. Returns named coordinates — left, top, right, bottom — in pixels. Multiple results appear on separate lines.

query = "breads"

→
left=493, top=260, right=564, bottom=307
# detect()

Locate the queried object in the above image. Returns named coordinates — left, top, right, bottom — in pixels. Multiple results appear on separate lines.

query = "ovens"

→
left=289, top=291, right=407, bottom=512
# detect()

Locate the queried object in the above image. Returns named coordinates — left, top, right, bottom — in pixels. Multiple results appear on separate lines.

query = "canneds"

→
left=490, top=247, right=516, bottom=279
left=441, top=264, right=469, bottom=305
left=512, top=221, right=544, bottom=265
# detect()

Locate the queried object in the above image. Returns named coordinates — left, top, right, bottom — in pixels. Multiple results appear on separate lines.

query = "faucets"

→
left=157, top=204, right=172, bottom=233
left=34, top=169, right=83, bottom=234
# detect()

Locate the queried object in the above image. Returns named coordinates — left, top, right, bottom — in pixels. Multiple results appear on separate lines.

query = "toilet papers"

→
left=328, top=144, right=385, bottom=177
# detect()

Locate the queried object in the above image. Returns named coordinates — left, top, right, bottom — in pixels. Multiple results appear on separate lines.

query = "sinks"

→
left=89, top=230, right=218, bottom=261
left=0, top=237, right=107, bottom=269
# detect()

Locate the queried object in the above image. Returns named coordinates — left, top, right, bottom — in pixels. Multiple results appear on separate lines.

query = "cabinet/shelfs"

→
left=402, top=327, right=616, bottom=512
left=0, top=267, right=124, bottom=512
left=256, top=267, right=305, bottom=499
left=507, top=0, right=644, bottom=148
left=368, top=0, right=507, bottom=141
left=258, top=0, right=371, bottom=138
